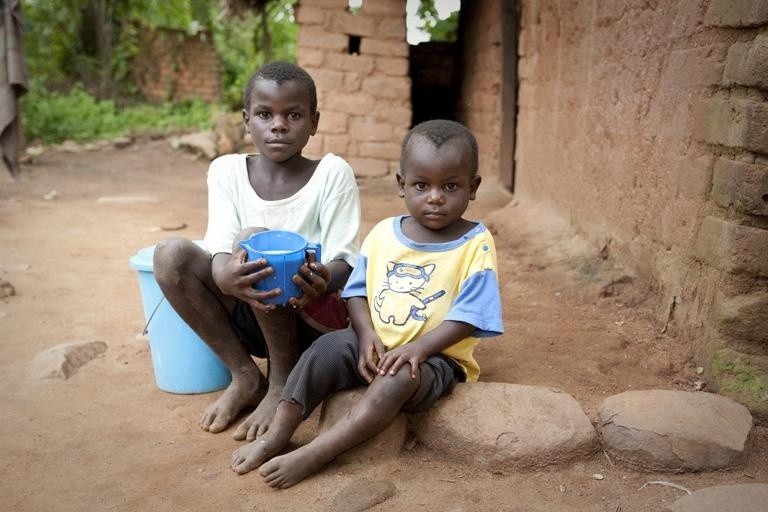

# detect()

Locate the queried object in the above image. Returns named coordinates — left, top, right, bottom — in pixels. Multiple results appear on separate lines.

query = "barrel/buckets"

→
left=129, top=241, right=232, bottom=395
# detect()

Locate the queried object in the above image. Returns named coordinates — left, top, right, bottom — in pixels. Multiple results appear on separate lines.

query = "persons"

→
left=152, top=61, right=361, bottom=442
left=232, top=119, right=502, bottom=490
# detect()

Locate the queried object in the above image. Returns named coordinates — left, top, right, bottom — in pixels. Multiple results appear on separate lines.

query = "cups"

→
left=238, top=229, right=322, bottom=306
